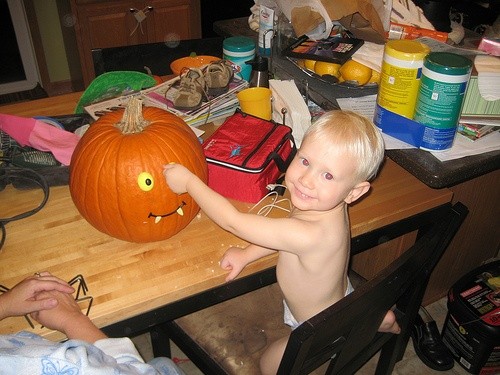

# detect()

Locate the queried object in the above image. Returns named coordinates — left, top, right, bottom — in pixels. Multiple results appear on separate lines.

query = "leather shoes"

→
left=410, top=305, right=454, bottom=371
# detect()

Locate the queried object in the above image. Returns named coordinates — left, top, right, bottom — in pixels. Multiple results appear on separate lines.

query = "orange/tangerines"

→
left=298, top=58, right=381, bottom=86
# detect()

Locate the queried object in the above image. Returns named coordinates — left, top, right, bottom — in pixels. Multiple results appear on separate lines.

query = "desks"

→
left=215, top=18, right=500, bottom=300
left=0, top=74, right=454, bottom=344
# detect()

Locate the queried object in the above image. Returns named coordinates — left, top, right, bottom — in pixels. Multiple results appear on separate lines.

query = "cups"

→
left=237, top=87, right=272, bottom=121
left=222, top=38, right=255, bottom=82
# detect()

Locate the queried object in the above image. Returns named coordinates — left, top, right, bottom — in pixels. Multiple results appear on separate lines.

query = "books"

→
left=458, top=123, right=500, bottom=142
left=86, top=63, right=248, bottom=132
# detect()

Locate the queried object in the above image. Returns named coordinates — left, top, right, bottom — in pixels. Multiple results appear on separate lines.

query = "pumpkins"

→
left=68, top=95, right=208, bottom=242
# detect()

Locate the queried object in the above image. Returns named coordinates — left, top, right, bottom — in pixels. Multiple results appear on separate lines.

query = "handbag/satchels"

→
left=203, top=109, right=298, bottom=203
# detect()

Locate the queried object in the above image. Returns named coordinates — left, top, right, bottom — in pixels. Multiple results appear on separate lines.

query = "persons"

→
left=161, top=109, right=404, bottom=375
left=0, top=271, right=185, bottom=375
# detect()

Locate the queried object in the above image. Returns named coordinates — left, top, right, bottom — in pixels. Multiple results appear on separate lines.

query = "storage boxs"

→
left=441, top=260, right=500, bottom=375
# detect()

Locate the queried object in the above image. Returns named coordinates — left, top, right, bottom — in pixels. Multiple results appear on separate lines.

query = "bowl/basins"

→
left=170, top=55, right=221, bottom=76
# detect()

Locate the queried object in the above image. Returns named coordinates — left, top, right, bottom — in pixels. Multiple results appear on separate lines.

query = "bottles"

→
left=412, top=51, right=477, bottom=150
left=376, top=41, right=429, bottom=134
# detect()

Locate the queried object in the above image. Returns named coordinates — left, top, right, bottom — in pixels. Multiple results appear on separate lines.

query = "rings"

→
left=33, top=272, right=41, bottom=277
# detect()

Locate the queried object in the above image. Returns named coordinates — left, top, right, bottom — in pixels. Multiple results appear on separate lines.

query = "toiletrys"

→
left=257, top=4, right=275, bottom=57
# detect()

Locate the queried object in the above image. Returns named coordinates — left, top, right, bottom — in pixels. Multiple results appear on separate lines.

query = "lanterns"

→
left=67, top=98, right=207, bottom=243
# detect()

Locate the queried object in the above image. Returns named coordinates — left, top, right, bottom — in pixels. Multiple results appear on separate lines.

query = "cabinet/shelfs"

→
left=55, top=0, right=202, bottom=92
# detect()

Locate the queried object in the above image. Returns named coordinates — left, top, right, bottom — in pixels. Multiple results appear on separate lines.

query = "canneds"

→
left=412, top=52, right=473, bottom=130
left=376, top=39, right=432, bottom=120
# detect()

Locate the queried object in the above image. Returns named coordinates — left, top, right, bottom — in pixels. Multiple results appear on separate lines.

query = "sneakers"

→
left=205, top=60, right=244, bottom=95
left=164, top=66, right=211, bottom=125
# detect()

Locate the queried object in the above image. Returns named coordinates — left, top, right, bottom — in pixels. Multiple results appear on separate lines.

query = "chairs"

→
left=149, top=202, right=470, bottom=375
